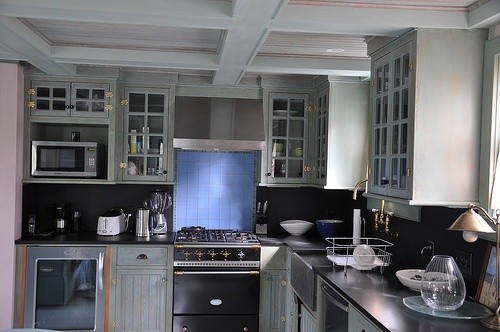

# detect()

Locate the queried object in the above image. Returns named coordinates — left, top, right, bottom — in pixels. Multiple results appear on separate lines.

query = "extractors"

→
left=173, top=96, right=265, bottom=153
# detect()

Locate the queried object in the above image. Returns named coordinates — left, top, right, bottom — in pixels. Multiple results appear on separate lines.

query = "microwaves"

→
left=29, top=140, right=106, bottom=178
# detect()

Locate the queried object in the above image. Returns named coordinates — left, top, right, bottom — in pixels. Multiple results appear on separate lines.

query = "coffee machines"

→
left=57, top=201, right=69, bottom=234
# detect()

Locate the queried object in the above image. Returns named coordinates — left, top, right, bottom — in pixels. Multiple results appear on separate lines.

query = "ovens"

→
left=173, top=270, right=259, bottom=332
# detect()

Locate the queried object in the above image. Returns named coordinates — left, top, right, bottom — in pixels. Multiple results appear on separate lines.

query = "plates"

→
left=353, top=244, right=376, bottom=265
left=274, top=110, right=299, bottom=115
left=403, top=296, right=493, bottom=319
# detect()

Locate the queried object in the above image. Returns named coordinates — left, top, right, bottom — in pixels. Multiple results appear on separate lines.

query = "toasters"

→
left=97, top=209, right=125, bottom=235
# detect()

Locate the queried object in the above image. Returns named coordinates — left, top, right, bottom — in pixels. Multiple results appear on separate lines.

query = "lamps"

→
left=446, top=204, right=500, bottom=330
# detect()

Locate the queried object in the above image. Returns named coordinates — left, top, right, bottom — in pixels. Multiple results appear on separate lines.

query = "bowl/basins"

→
left=317, top=220, right=344, bottom=239
left=271, top=143, right=285, bottom=157
left=396, top=269, right=457, bottom=295
left=148, top=149, right=159, bottom=154
left=279, top=220, right=315, bottom=236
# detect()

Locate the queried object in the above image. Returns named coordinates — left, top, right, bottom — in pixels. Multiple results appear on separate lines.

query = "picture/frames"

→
left=474, top=242, right=498, bottom=311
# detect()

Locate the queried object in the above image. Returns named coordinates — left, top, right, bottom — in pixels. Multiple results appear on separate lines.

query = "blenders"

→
left=144, top=188, right=173, bottom=235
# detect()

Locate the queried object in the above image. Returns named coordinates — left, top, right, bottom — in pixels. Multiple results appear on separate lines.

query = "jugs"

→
left=136, top=208, right=153, bottom=237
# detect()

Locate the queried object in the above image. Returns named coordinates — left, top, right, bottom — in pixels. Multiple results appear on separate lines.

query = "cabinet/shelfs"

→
left=108, top=244, right=174, bottom=332
left=255, top=27, right=488, bottom=208
left=259, top=246, right=383, bottom=332
left=21, top=75, right=176, bottom=186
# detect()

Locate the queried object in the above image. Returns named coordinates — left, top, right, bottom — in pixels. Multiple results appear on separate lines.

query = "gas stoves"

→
left=174, top=225, right=260, bottom=267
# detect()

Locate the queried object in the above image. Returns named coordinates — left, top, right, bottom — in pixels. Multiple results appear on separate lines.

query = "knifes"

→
left=257, top=201, right=267, bottom=214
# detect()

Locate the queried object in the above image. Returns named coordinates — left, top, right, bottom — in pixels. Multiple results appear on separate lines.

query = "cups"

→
left=148, top=168, right=154, bottom=175
